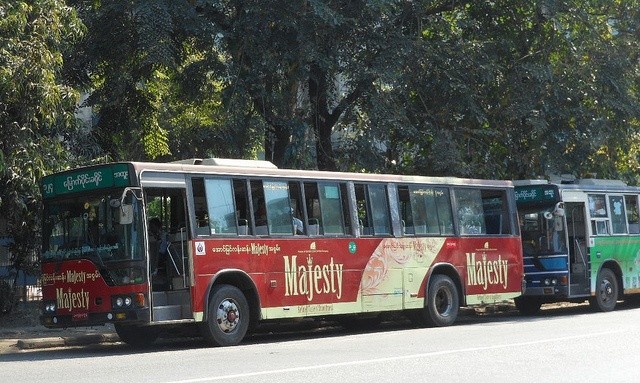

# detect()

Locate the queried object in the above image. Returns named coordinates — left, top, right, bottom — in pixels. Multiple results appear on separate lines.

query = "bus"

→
left=510, top=175, right=639, bottom=312
left=35, top=155, right=527, bottom=345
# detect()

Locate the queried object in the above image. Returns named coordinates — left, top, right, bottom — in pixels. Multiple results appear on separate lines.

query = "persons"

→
left=149, top=218, right=170, bottom=257
left=293, top=217, right=303, bottom=235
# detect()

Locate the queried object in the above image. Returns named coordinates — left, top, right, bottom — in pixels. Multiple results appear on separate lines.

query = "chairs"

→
left=272, top=218, right=296, bottom=234
left=345, top=217, right=363, bottom=235
left=307, top=217, right=320, bottom=235
left=174, top=218, right=200, bottom=242
left=385, top=218, right=407, bottom=236
left=463, top=222, right=482, bottom=235
left=252, top=218, right=271, bottom=234
left=427, top=220, right=445, bottom=235
left=446, top=224, right=464, bottom=234
left=221, top=218, right=249, bottom=236
left=406, top=220, right=427, bottom=235
